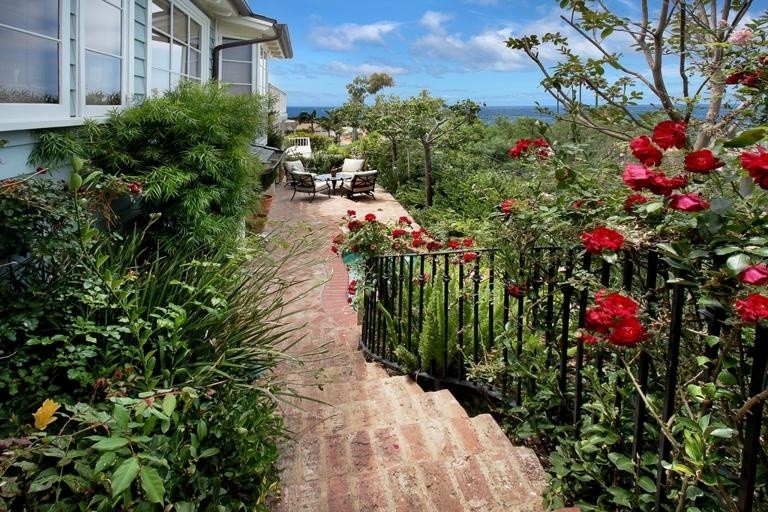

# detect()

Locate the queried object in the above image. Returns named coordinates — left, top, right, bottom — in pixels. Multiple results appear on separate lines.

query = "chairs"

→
left=289, top=170, right=332, bottom=204
left=333, top=158, right=365, bottom=173
left=340, top=170, right=377, bottom=201
left=282, top=159, right=317, bottom=188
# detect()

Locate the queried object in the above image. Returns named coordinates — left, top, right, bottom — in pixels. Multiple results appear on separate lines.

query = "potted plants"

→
left=329, top=155, right=342, bottom=177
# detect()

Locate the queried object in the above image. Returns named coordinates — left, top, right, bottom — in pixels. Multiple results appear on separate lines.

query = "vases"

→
left=244, top=214, right=269, bottom=236
left=257, top=194, right=272, bottom=217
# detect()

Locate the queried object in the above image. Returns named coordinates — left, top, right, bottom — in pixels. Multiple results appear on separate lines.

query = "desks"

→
left=314, top=173, right=352, bottom=196
